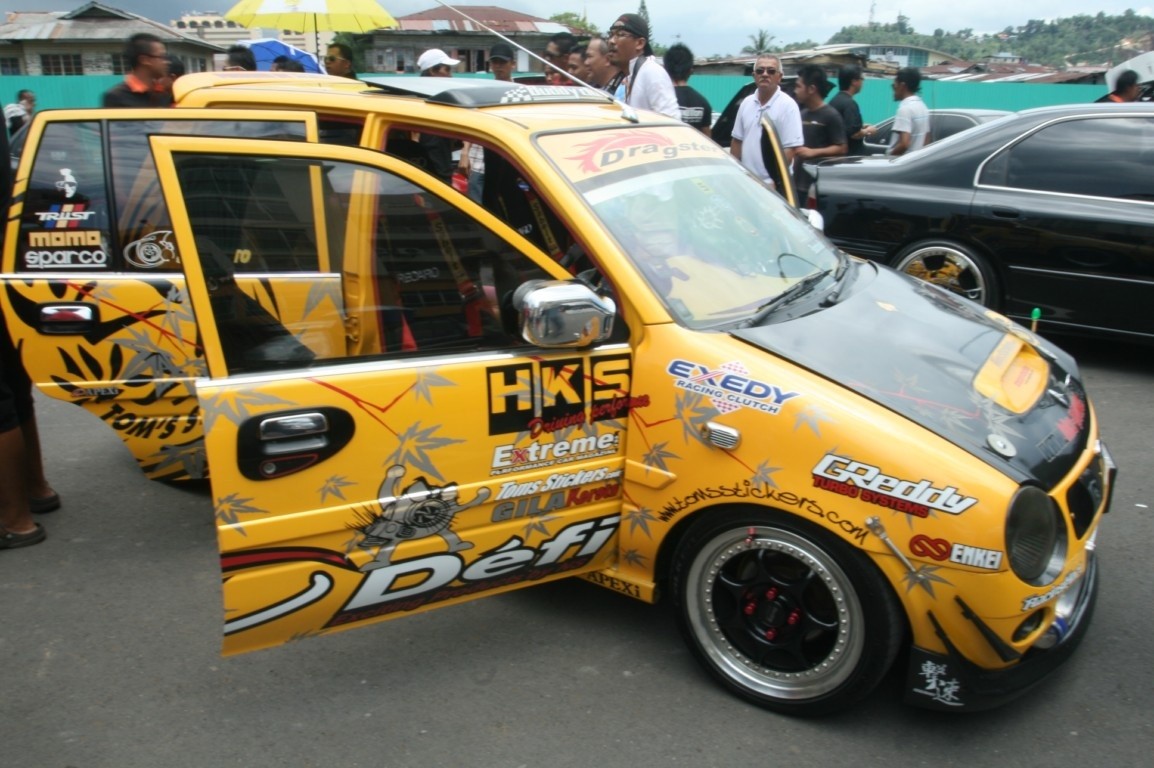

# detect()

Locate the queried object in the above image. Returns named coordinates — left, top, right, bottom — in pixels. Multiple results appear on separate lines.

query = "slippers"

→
left=0, top=523, right=46, bottom=550
left=31, top=485, right=58, bottom=512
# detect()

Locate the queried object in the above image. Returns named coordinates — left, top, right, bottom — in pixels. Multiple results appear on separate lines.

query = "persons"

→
left=0, top=106, right=60, bottom=548
left=9, top=89, right=35, bottom=136
left=792, top=66, right=848, bottom=208
left=269, top=56, right=304, bottom=72
left=663, top=45, right=711, bottom=138
left=326, top=43, right=356, bottom=79
left=828, top=62, right=877, bottom=154
left=607, top=14, right=678, bottom=121
left=1103, top=71, right=1139, bottom=102
left=887, top=67, right=930, bottom=158
left=458, top=44, right=515, bottom=205
left=731, top=54, right=804, bottom=189
left=100, top=32, right=185, bottom=211
left=221, top=46, right=257, bottom=72
left=542, top=32, right=624, bottom=102
left=399, top=49, right=461, bottom=177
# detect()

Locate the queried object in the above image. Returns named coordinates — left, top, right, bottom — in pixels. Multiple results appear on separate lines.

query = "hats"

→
left=417, top=48, right=460, bottom=72
left=488, top=44, right=514, bottom=62
left=611, top=13, right=655, bottom=55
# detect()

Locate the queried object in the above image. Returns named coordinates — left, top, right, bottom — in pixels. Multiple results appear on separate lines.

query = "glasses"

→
left=223, top=65, right=246, bottom=71
left=143, top=52, right=168, bottom=61
left=324, top=55, right=349, bottom=63
left=433, top=66, right=451, bottom=72
left=544, top=50, right=561, bottom=59
left=608, top=30, right=640, bottom=39
left=753, top=69, right=781, bottom=75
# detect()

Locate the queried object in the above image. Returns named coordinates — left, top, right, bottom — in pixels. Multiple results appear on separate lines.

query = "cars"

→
left=806, top=101, right=1149, bottom=351
left=1, top=69, right=1117, bottom=716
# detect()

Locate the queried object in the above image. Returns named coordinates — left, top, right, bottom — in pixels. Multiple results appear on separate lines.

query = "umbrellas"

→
left=238, top=39, right=324, bottom=73
left=223, top=0, right=400, bottom=73
